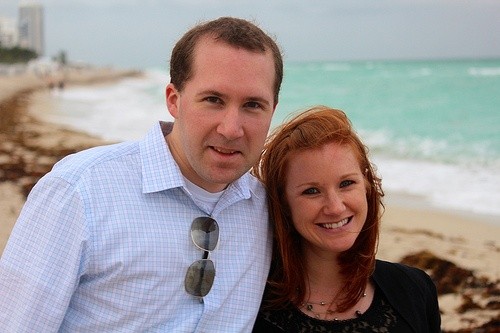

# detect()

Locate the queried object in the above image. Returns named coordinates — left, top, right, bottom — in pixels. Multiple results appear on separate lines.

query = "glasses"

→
left=184, top=217, right=218, bottom=304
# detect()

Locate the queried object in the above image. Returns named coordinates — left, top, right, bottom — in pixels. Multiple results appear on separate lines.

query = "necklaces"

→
left=296, top=271, right=367, bottom=333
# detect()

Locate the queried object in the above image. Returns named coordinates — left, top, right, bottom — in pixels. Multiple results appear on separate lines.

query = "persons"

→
left=250, top=105, right=440, bottom=333
left=0, top=17, right=283, bottom=333
